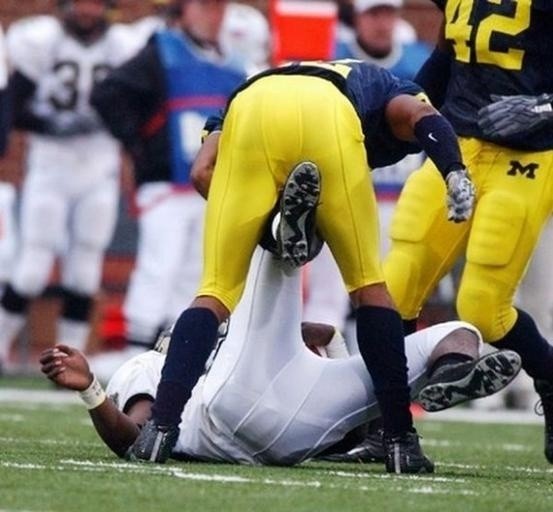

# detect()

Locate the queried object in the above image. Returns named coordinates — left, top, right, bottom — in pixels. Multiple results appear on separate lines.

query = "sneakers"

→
left=417, top=348, right=524, bottom=413
left=531, top=344, right=553, bottom=467
left=273, top=158, right=324, bottom=273
left=383, top=418, right=436, bottom=478
left=123, top=417, right=181, bottom=465
left=312, top=422, right=387, bottom=465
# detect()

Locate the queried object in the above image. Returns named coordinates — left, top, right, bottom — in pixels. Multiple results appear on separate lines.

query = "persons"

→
left=1, top=2, right=553, bottom=473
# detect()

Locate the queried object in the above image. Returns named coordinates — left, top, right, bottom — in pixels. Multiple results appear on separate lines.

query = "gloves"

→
left=473, top=92, right=553, bottom=142
left=440, top=161, right=483, bottom=234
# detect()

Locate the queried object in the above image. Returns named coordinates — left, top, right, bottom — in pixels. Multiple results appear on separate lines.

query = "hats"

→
left=351, top=0, right=404, bottom=15
